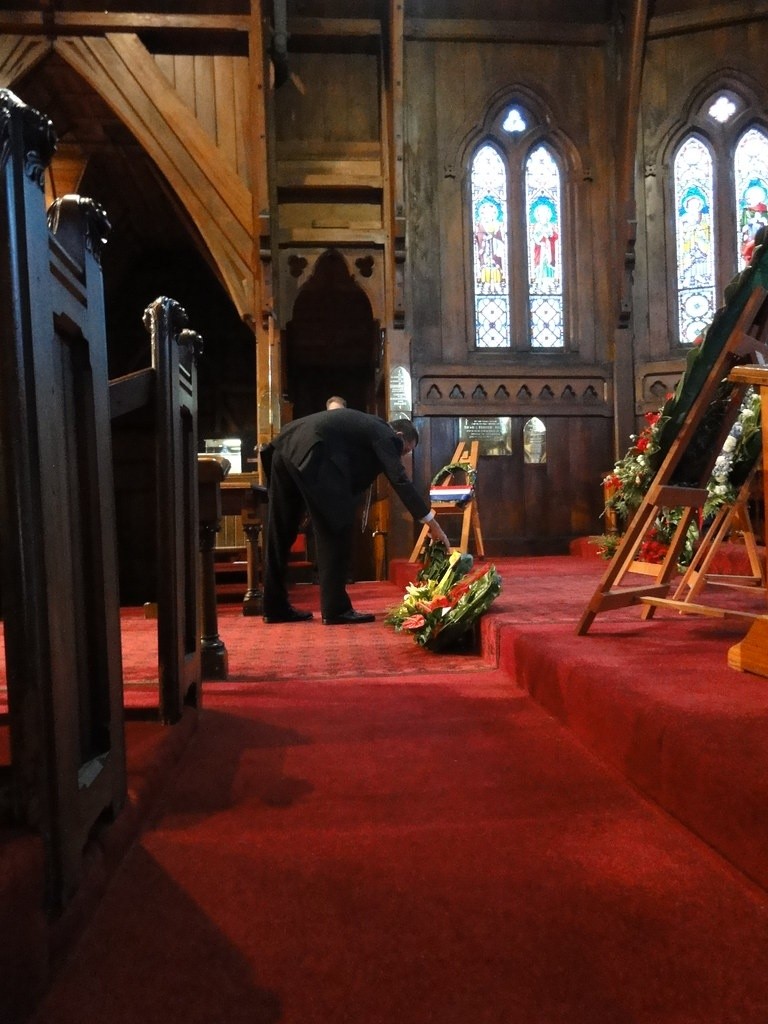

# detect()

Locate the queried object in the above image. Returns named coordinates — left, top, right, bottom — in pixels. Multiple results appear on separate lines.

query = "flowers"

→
left=588, top=224, right=768, bottom=575
left=382, top=542, right=502, bottom=650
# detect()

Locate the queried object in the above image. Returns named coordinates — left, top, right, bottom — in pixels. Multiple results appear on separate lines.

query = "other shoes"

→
left=345, top=578, right=354, bottom=584
left=312, top=579, right=320, bottom=585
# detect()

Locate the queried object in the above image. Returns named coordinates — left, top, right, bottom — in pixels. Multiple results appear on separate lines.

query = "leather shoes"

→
left=263, top=607, right=313, bottom=623
left=322, top=609, right=375, bottom=625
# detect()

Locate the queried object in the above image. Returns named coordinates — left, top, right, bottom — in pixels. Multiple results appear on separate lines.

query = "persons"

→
left=326, top=396, right=347, bottom=410
left=261, top=408, right=450, bottom=625
